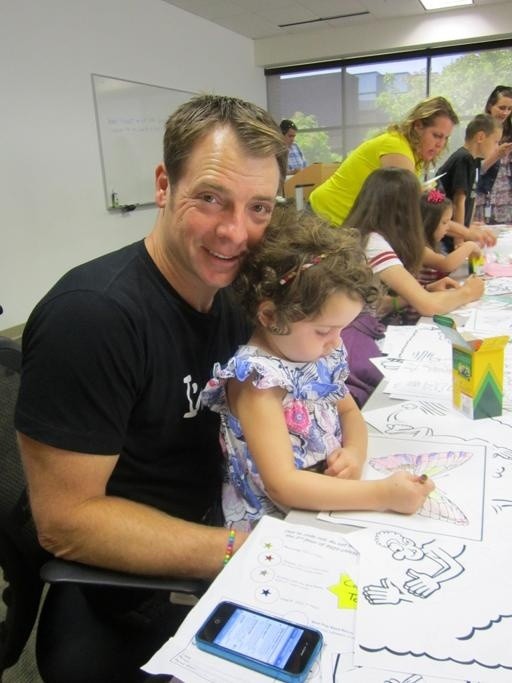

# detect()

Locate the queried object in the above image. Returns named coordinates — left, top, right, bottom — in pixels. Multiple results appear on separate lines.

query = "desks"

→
left=144, top=217, right=512, bottom=682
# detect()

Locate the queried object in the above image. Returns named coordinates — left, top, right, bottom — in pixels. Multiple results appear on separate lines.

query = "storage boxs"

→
left=430, top=312, right=508, bottom=420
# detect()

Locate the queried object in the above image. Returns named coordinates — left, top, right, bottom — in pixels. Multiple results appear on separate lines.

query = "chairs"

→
left=0, top=320, right=205, bottom=683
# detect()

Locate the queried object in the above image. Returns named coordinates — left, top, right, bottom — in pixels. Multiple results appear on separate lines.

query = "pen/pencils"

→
left=419, top=474, right=427, bottom=484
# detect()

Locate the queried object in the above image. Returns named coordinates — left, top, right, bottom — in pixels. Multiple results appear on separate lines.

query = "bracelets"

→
left=224, top=529, right=234, bottom=566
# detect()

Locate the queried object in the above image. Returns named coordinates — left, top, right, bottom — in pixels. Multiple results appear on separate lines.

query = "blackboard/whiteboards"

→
left=91, top=73, right=201, bottom=210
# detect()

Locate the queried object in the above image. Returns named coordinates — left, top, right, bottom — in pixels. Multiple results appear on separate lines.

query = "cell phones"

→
left=195, top=601, right=323, bottom=683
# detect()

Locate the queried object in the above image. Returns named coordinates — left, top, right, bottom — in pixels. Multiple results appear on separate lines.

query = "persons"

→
left=200, top=207, right=436, bottom=533
left=278, top=119, right=306, bottom=175
left=19, top=98, right=290, bottom=682
left=304, top=86, right=512, bottom=314
